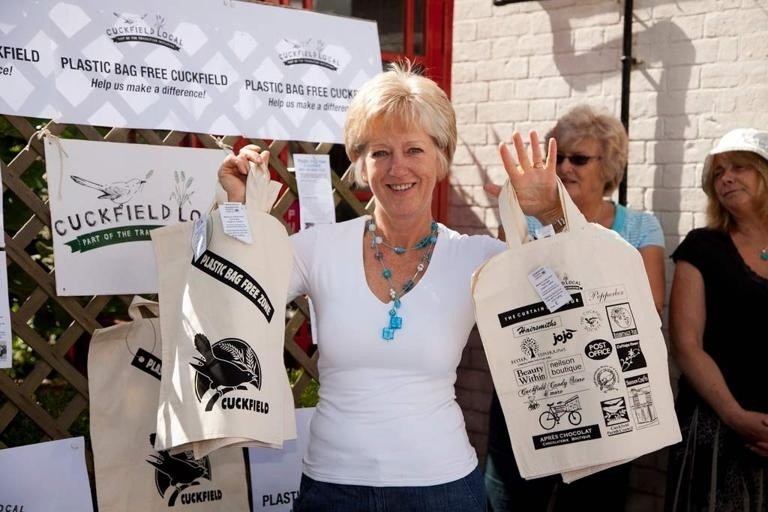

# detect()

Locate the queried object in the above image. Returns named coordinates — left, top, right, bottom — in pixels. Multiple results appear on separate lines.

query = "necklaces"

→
left=756, top=244, right=767, bottom=261
left=371, top=219, right=435, bottom=254
left=364, top=211, right=437, bottom=339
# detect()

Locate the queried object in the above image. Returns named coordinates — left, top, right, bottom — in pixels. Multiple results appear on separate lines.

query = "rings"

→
left=534, top=162, right=543, bottom=169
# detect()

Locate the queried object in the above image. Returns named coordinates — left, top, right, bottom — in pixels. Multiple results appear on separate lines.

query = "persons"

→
left=660, top=125, right=766, bottom=511
left=216, top=56, right=566, bottom=511
left=484, top=106, right=667, bottom=507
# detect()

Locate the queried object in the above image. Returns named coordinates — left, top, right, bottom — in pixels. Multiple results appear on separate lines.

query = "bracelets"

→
left=551, top=217, right=566, bottom=233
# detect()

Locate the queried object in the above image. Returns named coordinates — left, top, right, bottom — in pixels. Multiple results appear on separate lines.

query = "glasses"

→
left=556, top=154, right=600, bottom=165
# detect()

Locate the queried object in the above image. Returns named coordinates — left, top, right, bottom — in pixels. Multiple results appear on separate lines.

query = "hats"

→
left=701, top=128, right=768, bottom=192
